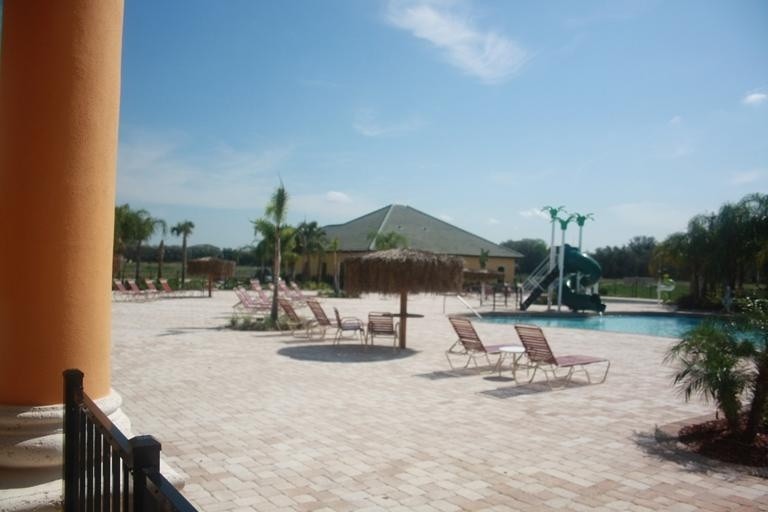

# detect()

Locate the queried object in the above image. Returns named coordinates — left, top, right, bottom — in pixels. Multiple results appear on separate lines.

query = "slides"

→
left=556, top=244, right=606, bottom=313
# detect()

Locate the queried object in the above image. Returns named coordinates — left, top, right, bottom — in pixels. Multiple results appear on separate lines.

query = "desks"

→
left=497, top=344, right=530, bottom=381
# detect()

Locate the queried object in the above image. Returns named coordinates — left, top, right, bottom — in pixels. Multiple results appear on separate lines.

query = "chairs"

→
left=331, top=307, right=368, bottom=350
left=111, top=276, right=178, bottom=302
left=367, top=311, right=402, bottom=354
left=513, top=322, right=611, bottom=392
left=446, top=316, right=521, bottom=372
left=304, top=294, right=337, bottom=341
left=231, top=276, right=319, bottom=338
